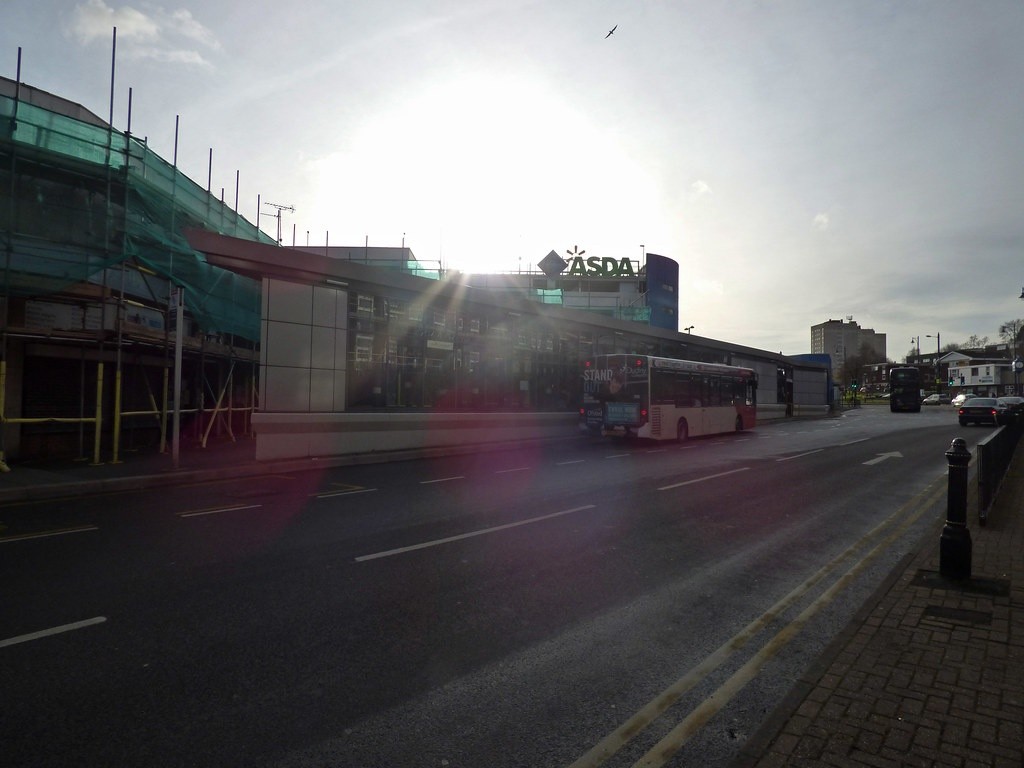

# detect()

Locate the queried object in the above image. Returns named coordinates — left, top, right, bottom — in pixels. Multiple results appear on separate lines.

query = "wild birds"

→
left=605, top=25, right=618, bottom=39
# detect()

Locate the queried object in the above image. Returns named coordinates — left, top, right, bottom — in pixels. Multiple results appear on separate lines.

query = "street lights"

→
left=926, top=332, right=941, bottom=393
left=1000, top=322, right=1017, bottom=394
left=684, top=326, right=694, bottom=334
left=640, top=244, right=645, bottom=271
left=911, top=336, right=920, bottom=365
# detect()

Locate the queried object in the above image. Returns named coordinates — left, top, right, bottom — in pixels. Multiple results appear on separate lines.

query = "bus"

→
left=889, top=367, right=923, bottom=415
left=581, top=353, right=759, bottom=446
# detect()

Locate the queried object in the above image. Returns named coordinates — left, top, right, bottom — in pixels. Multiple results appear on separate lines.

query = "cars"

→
left=959, top=397, right=1009, bottom=426
left=922, top=393, right=950, bottom=406
left=998, top=397, right=1023, bottom=417
left=951, top=393, right=977, bottom=406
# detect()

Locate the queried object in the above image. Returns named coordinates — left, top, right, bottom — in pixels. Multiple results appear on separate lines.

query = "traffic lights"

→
left=961, top=377, right=965, bottom=385
left=852, top=381, right=854, bottom=389
left=950, top=377, right=953, bottom=385
left=854, top=380, right=857, bottom=390
